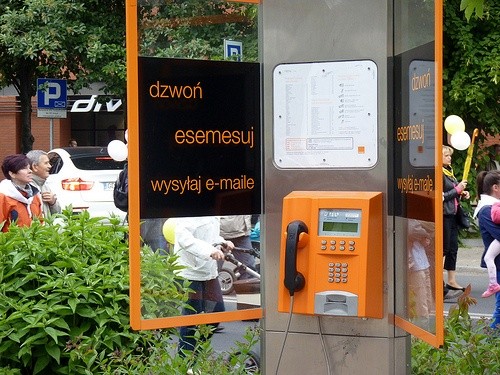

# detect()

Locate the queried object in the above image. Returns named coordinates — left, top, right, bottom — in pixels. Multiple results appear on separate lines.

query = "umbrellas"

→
left=459, top=128, right=478, bottom=199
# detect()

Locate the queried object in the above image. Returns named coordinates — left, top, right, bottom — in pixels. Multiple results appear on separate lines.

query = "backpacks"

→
left=113, top=161, right=128, bottom=212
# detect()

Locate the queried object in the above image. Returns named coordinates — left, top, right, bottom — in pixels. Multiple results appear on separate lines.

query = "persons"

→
left=441, top=145, right=468, bottom=293
left=68, top=140, right=77, bottom=148
left=472, top=170, right=500, bottom=338
left=0, top=153, right=44, bottom=236
left=139, top=219, right=172, bottom=260
left=406, top=217, right=435, bottom=323
left=421, top=221, right=464, bottom=301
left=480, top=202, right=500, bottom=299
left=174, top=216, right=236, bottom=375
left=216, top=216, right=256, bottom=281
left=25, top=150, right=63, bottom=227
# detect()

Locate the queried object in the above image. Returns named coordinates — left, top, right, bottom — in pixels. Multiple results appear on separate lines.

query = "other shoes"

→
left=447, top=284, right=466, bottom=292
left=443, top=287, right=464, bottom=300
left=482, top=282, right=500, bottom=298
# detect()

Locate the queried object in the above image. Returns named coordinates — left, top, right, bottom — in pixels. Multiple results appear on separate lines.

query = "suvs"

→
left=43, top=147, right=127, bottom=228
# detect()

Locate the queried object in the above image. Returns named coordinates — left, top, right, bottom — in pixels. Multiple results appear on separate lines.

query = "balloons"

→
left=162, top=218, right=174, bottom=245
left=449, top=131, right=471, bottom=151
left=444, top=115, right=465, bottom=135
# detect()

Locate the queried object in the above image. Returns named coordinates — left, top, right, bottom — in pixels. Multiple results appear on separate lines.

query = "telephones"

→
left=276, top=190, right=383, bottom=319
